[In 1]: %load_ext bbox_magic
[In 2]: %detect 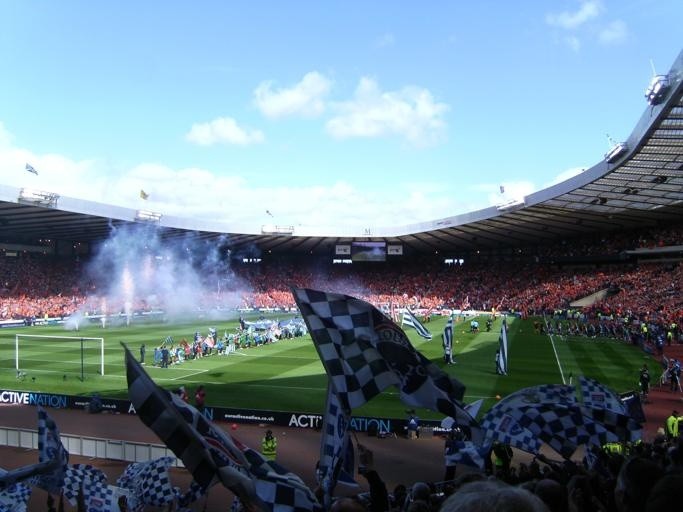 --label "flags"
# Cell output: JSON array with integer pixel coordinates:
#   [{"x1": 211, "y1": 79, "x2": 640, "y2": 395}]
[
  {"x1": 500, "y1": 186, "x2": 505, "y2": 193},
  {"x1": 140, "y1": 189, "x2": 149, "y2": 201},
  {"x1": 25, "y1": 162, "x2": 39, "y2": 177}
]
